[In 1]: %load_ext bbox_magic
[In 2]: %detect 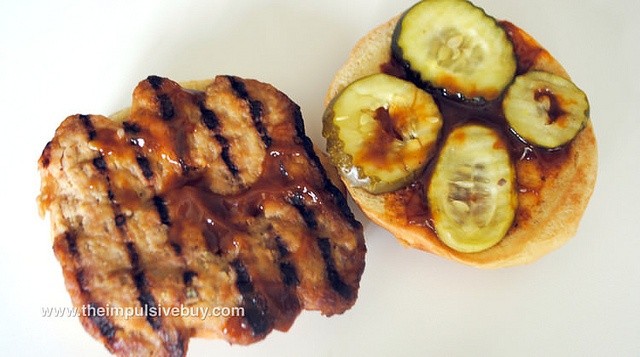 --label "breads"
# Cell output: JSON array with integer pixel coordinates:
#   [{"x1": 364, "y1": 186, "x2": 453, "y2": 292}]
[
  {"x1": 37, "y1": 77, "x2": 367, "y2": 357},
  {"x1": 322, "y1": 13, "x2": 599, "y2": 271}
]
